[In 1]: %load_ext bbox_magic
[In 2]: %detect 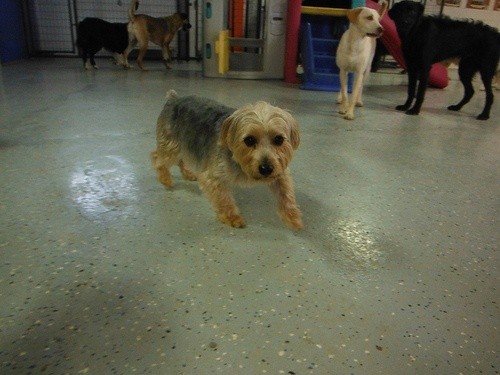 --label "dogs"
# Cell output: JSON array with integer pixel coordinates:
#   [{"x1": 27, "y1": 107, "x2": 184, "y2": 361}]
[
  {"x1": 75, "y1": 0, "x2": 192, "y2": 71},
  {"x1": 387, "y1": 0, "x2": 500, "y2": 120},
  {"x1": 152, "y1": 88, "x2": 304, "y2": 231},
  {"x1": 335, "y1": 6, "x2": 383, "y2": 120}
]
